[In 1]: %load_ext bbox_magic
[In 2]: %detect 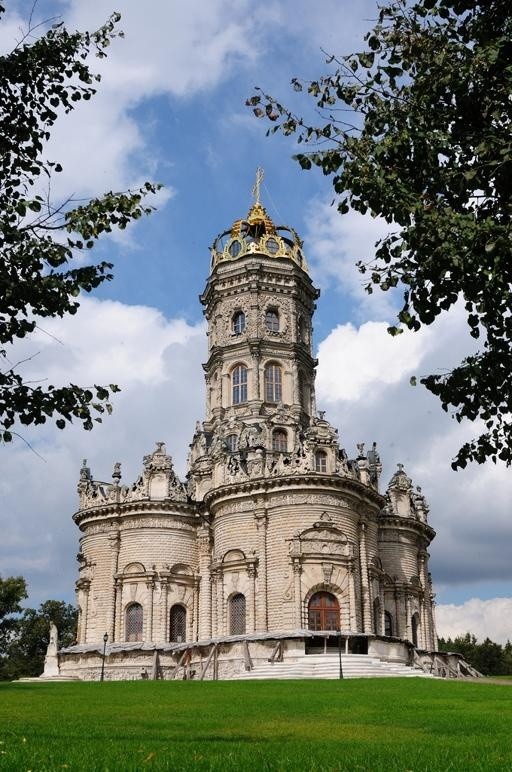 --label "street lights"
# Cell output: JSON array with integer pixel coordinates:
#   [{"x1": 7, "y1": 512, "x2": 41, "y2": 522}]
[
  {"x1": 99, "y1": 632, "x2": 109, "y2": 681},
  {"x1": 336, "y1": 625, "x2": 345, "y2": 680}
]
